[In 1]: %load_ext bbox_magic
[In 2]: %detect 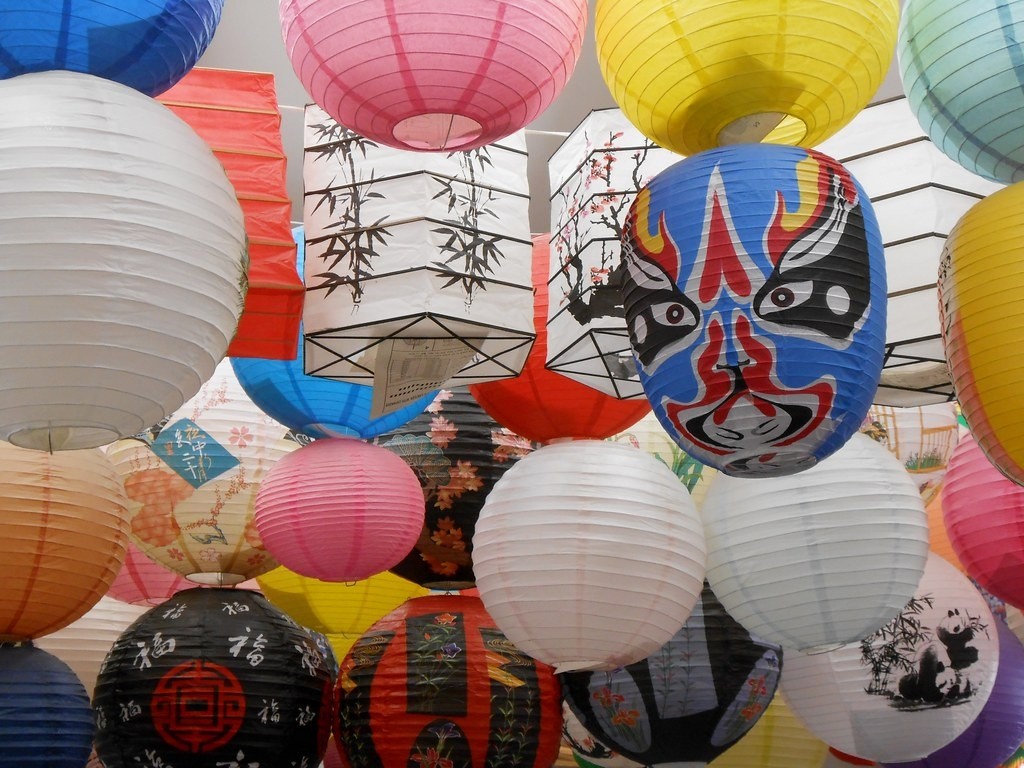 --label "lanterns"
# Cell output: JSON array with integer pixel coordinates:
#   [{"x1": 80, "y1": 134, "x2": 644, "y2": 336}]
[
  {"x1": 365, "y1": 385, "x2": 541, "y2": 590},
  {"x1": 809, "y1": 94, "x2": 1009, "y2": 407},
  {"x1": 0, "y1": 441, "x2": 130, "y2": 642},
  {"x1": 229, "y1": 223, "x2": 441, "y2": 440},
  {"x1": 0, "y1": 0, "x2": 223, "y2": 98},
  {"x1": 622, "y1": 144, "x2": 886, "y2": 477},
  {"x1": 941, "y1": 427, "x2": 1024, "y2": 612},
  {"x1": 858, "y1": 404, "x2": 957, "y2": 507},
  {"x1": 301, "y1": 103, "x2": 534, "y2": 391},
  {"x1": 603, "y1": 408, "x2": 716, "y2": 511},
  {"x1": 706, "y1": 431, "x2": 927, "y2": 658},
  {"x1": 898, "y1": 0, "x2": 1024, "y2": 184},
  {"x1": 0, "y1": 70, "x2": 247, "y2": 454},
  {"x1": 107, "y1": 354, "x2": 317, "y2": 586},
  {"x1": 548, "y1": 107, "x2": 687, "y2": 401},
  {"x1": 465, "y1": 232, "x2": 653, "y2": 443},
  {"x1": 276, "y1": 0, "x2": 590, "y2": 154},
  {"x1": 472, "y1": 439, "x2": 705, "y2": 673},
  {"x1": 155, "y1": 68, "x2": 306, "y2": 362},
  {"x1": 0, "y1": 492, "x2": 1024, "y2": 767},
  {"x1": 937, "y1": 175, "x2": 1024, "y2": 486},
  {"x1": 255, "y1": 436, "x2": 425, "y2": 587},
  {"x1": 596, "y1": 1, "x2": 898, "y2": 156}
]
